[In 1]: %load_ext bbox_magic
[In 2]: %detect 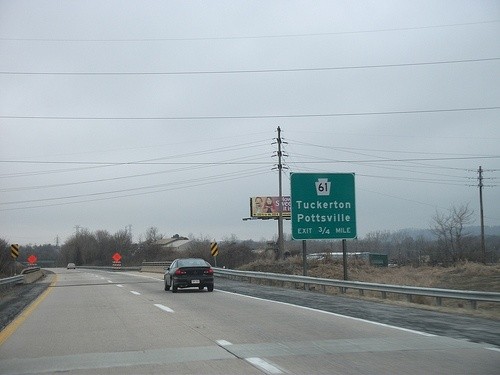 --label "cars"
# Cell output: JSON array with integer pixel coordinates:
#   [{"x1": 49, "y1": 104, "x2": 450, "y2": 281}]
[
  {"x1": 67, "y1": 263, "x2": 76, "y2": 269},
  {"x1": 164, "y1": 258, "x2": 215, "y2": 293}
]
